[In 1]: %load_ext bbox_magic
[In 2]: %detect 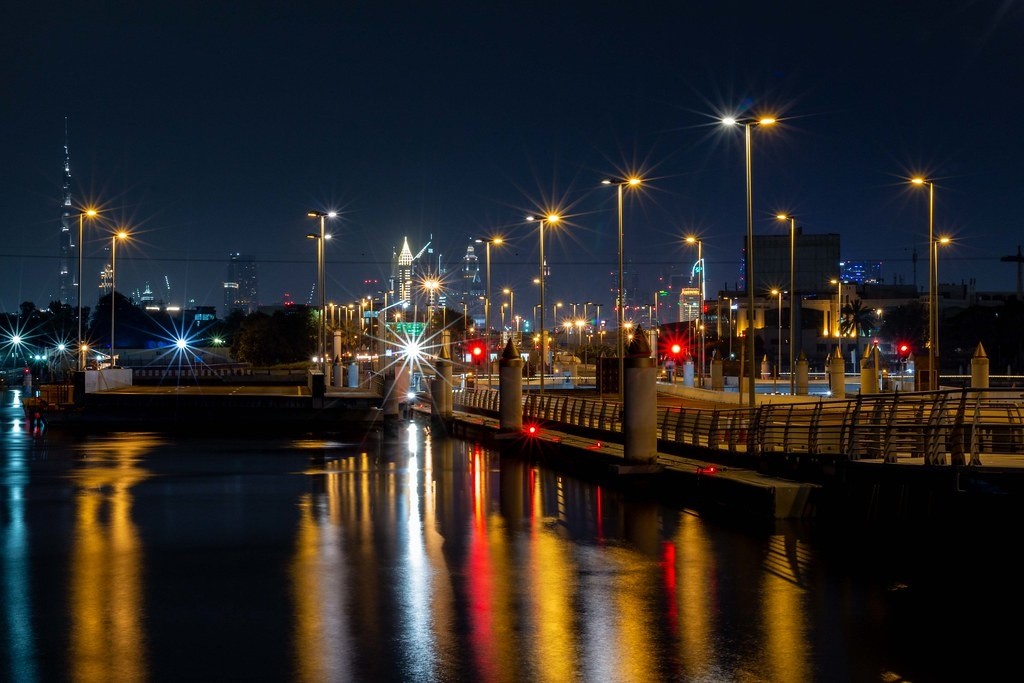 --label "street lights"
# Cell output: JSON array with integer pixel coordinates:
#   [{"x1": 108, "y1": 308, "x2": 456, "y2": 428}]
[
  {"x1": 423, "y1": 277, "x2": 442, "y2": 373},
  {"x1": 460, "y1": 286, "x2": 655, "y2": 344},
  {"x1": 831, "y1": 279, "x2": 842, "y2": 358},
  {"x1": 771, "y1": 290, "x2": 782, "y2": 391},
  {"x1": 476, "y1": 236, "x2": 505, "y2": 388},
  {"x1": 933, "y1": 236, "x2": 951, "y2": 391},
  {"x1": 525, "y1": 214, "x2": 562, "y2": 397},
  {"x1": 722, "y1": 111, "x2": 780, "y2": 412},
  {"x1": 777, "y1": 212, "x2": 796, "y2": 396},
  {"x1": 309, "y1": 209, "x2": 339, "y2": 386},
  {"x1": 307, "y1": 232, "x2": 331, "y2": 385},
  {"x1": 722, "y1": 296, "x2": 732, "y2": 362},
  {"x1": 912, "y1": 176, "x2": 933, "y2": 393},
  {"x1": 329, "y1": 279, "x2": 413, "y2": 392},
  {"x1": 603, "y1": 173, "x2": 644, "y2": 407}
]
